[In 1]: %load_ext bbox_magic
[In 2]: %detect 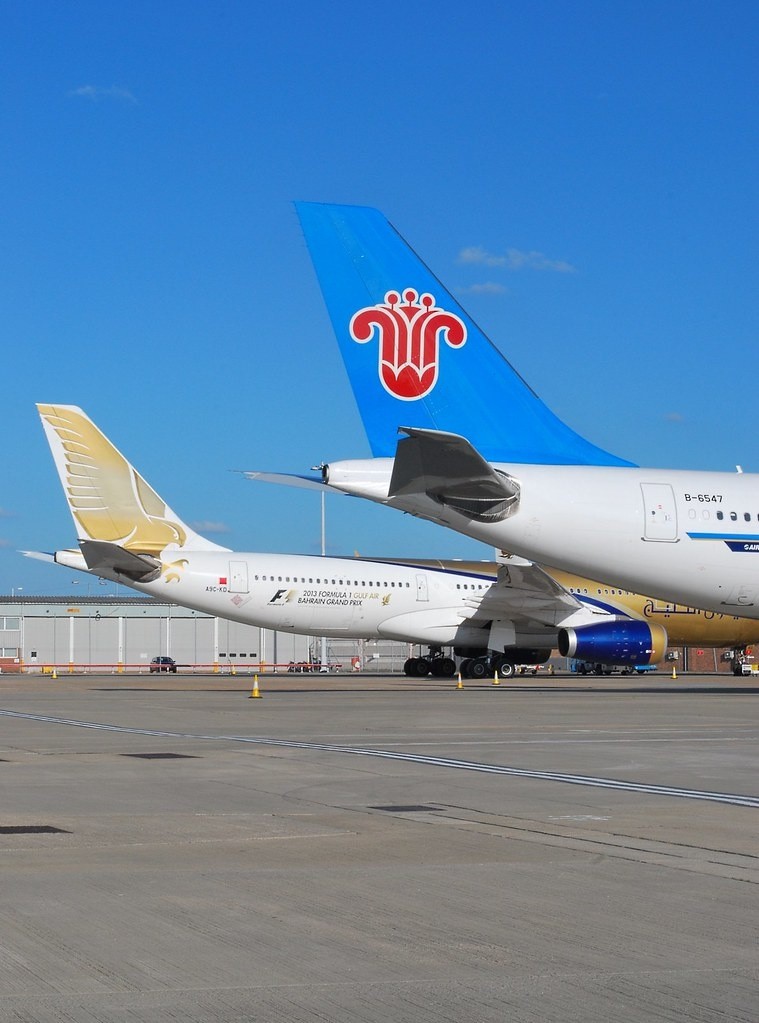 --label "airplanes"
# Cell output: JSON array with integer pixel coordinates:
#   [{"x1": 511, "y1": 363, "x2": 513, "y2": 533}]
[
  {"x1": 231, "y1": 200, "x2": 759, "y2": 620},
  {"x1": 14, "y1": 400, "x2": 759, "y2": 680}
]
